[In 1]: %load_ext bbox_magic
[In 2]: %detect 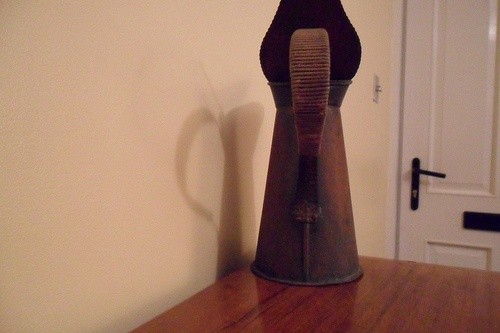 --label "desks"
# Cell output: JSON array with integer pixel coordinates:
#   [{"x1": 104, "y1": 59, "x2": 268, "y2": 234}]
[{"x1": 131, "y1": 254, "x2": 499, "y2": 333}]
